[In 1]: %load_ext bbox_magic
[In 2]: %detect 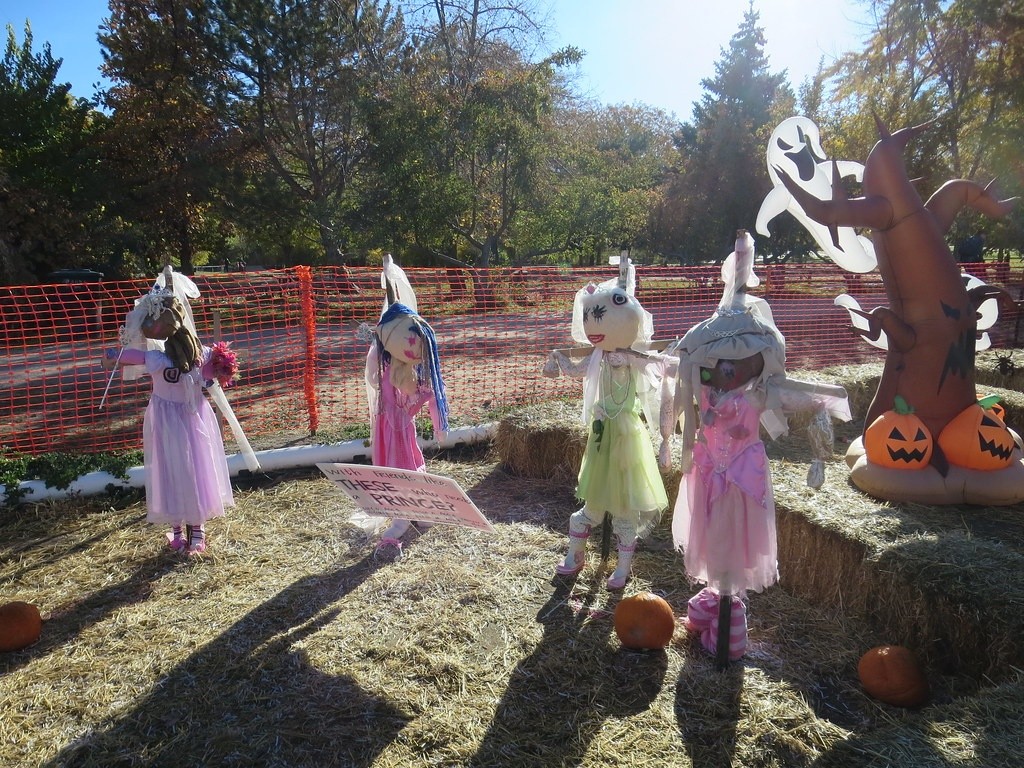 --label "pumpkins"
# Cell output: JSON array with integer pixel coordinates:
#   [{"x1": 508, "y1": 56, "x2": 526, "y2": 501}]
[
  {"x1": 0, "y1": 600, "x2": 42, "y2": 651},
  {"x1": 614, "y1": 591, "x2": 675, "y2": 649},
  {"x1": 857, "y1": 644, "x2": 925, "y2": 705}
]
